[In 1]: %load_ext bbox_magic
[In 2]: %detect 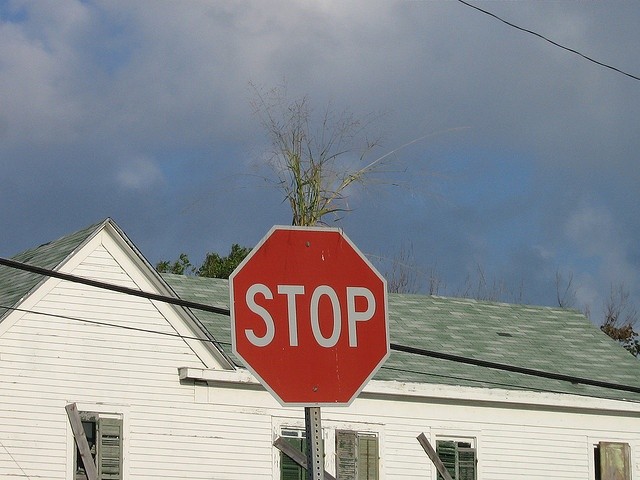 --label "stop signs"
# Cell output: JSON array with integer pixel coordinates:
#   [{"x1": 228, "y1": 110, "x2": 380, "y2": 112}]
[{"x1": 226, "y1": 224, "x2": 391, "y2": 409}]
